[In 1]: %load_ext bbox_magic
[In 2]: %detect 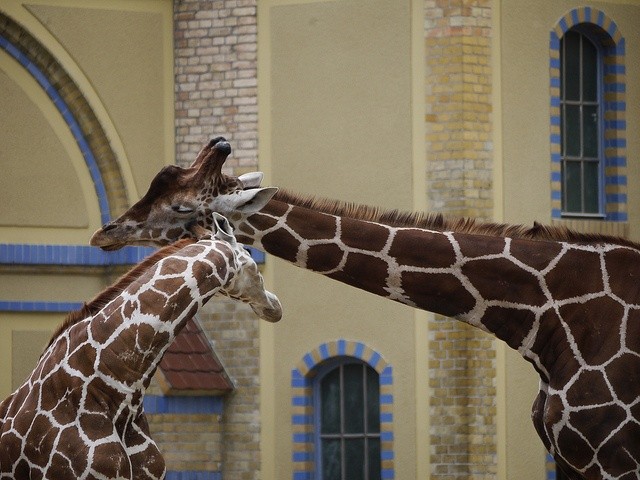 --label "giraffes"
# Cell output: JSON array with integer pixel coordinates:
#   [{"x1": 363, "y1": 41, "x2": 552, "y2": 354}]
[
  {"x1": 0, "y1": 210, "x2": 284, "y2": 479},
  {"x1": 88, "y1": 135, "x2": 639, "y2": 480}
]
